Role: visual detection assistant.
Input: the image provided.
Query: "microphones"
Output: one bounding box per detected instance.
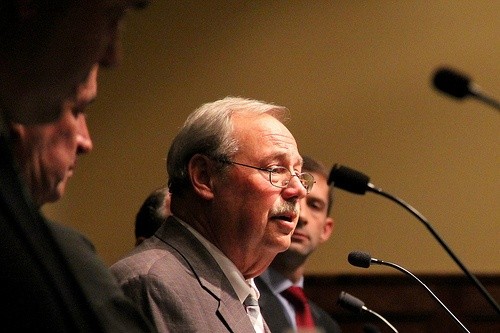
[336,290,400,333]
[432,66,500,114]
[347,249,471,333]
[326,163,500,314]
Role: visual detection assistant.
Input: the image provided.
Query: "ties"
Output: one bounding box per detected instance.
[279,286,316,333]
[243,287,265,333]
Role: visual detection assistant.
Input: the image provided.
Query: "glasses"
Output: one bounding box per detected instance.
[212,156,316,195]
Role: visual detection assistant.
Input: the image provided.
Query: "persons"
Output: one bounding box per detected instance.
[0,0,152,333]
[110,97,317,333]
[135,186,172,244]
[256,155,344,333]
